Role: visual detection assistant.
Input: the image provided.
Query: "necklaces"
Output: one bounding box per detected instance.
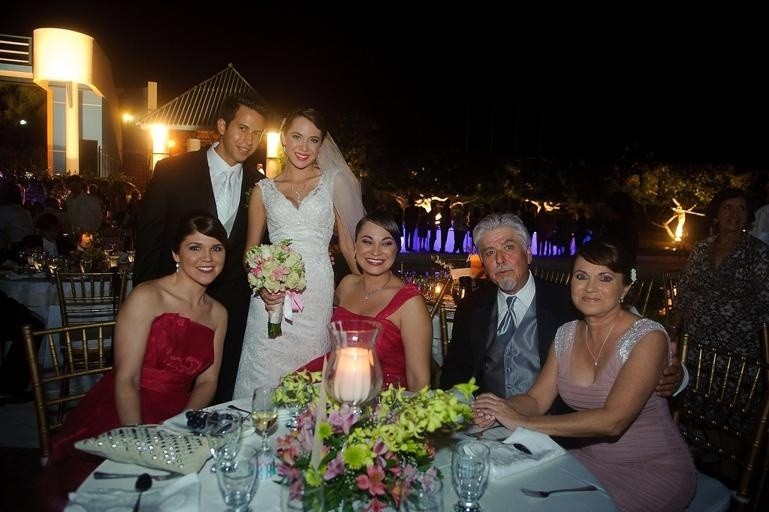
[585,318,617,367]
[362,275,391,299]
[284,176,309,204]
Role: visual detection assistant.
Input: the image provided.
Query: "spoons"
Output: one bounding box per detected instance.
[132,473,152,512]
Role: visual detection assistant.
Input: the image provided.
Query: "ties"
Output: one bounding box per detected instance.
[496,296,518,349]
[215,168,235,225]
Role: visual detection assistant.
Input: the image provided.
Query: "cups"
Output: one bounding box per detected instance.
[470,252,482,275]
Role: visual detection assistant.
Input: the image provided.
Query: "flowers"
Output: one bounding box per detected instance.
[245,239,306,339]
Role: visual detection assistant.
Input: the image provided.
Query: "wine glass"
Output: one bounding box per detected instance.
[205,386,278,512]
[280,470,325,512]
[451,439,491,512]
[400,274,463,305]
[16,250,27,274]
[27,250,136,277]
[399,471,446,511]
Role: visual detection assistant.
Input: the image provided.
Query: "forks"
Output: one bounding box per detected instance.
[521,485,599,499]
[94,471,183,482]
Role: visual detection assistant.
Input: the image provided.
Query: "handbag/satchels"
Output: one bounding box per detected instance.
[74,424,226,475]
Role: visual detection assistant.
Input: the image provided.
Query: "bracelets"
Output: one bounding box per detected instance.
[666,322,682,331]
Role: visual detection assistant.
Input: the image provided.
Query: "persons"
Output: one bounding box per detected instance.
[598,189,641,266]
[0,170,146,406]
[232,104,368,402]
[469,238,698,511]
[371,188,590,257]
[291,211,434,393]
[132,91,271,404]
[661,187,768,511]
[439,212,690,447]
[34,209,230,512]
